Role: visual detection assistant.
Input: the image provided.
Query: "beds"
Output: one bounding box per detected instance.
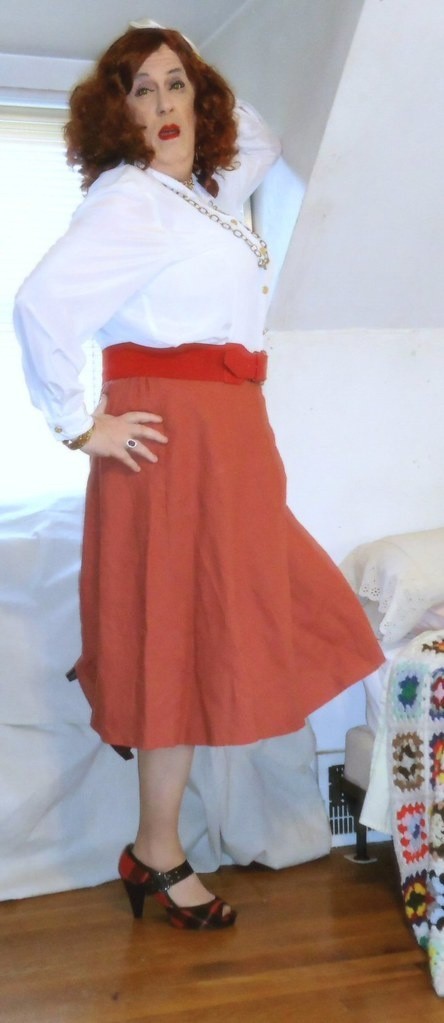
[341,629,444,999]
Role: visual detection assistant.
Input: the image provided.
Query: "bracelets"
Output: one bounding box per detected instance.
[63,424,94,451]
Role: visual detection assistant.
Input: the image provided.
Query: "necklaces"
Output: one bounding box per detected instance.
[161,183,269,269]
[181,179,194,189]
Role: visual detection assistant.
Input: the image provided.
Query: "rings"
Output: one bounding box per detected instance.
[127,439,137,449]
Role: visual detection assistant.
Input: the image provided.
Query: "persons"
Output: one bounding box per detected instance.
[12,20,386,930]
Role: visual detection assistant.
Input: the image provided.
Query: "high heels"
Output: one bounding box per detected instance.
[118,845,238,929]
[67,667,136,760]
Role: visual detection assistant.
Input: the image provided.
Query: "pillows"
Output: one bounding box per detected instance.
[338,529,444,646]
